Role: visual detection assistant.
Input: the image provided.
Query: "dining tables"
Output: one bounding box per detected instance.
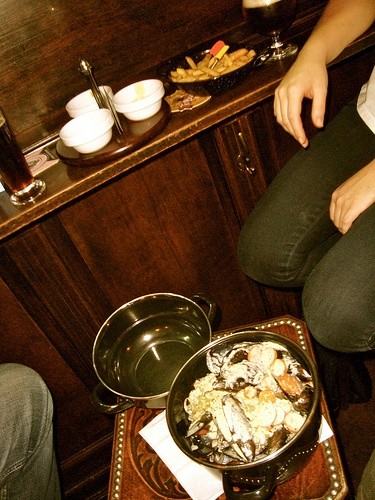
[104,314,349,500]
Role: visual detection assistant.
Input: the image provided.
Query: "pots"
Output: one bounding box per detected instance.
[87,292,217,415]
[155,41,270,97]
[166,327,321,500]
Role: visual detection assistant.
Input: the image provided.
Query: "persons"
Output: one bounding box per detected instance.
[238,0,375,352]
[0,363,62,500]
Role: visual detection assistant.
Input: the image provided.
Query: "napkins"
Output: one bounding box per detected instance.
[138,406,336,500]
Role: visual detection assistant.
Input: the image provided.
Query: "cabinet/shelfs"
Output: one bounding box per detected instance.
[0,4,375,500]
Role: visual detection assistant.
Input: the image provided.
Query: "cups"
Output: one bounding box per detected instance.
[0,105,46,205]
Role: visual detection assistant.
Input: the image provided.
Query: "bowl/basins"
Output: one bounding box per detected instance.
[112,79,165,121]
[65,85,114,118]
[58,109,115,154]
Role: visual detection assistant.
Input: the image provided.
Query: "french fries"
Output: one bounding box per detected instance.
[168,48,256,82]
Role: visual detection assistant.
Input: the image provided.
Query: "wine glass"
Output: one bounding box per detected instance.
[241,0,298,63]
